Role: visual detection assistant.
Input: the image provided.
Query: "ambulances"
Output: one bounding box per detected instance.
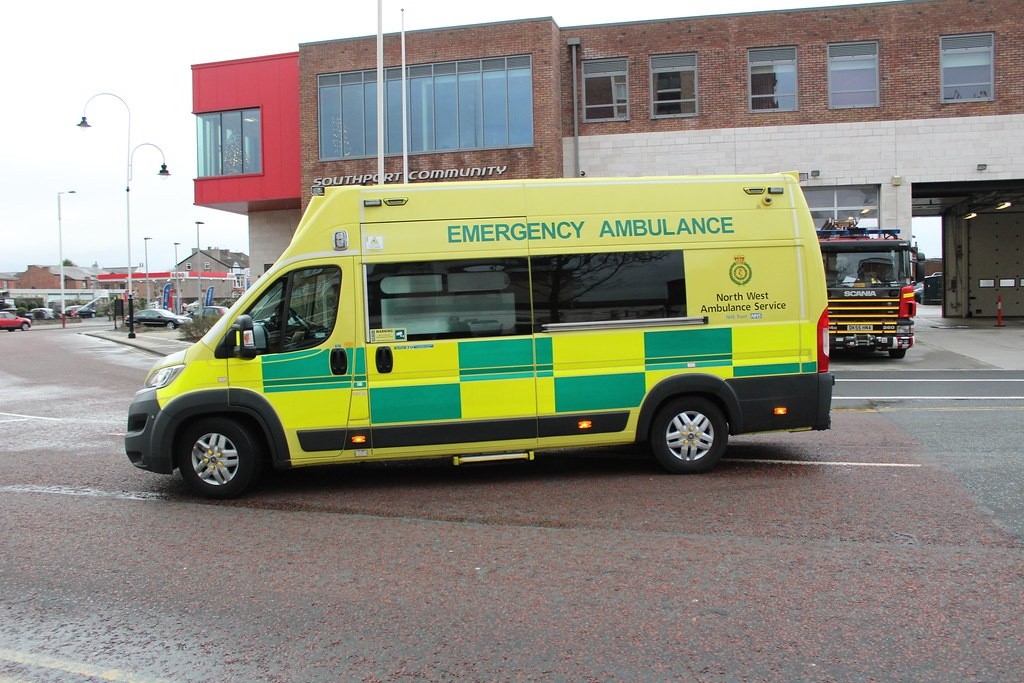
[124,171,834,497]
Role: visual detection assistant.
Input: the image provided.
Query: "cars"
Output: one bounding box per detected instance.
[125,309,193,331]
[915,272,942,303]
[0,312,31,332]
[63,305,96,318]
[30,308,55,320]
[921,276,942,305]
[181,306,230,324]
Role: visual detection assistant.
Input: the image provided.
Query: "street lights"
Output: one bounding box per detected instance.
[196,221,205,332]
[174,242,181,314]
[77,93,170,338]
[144,237,151,309]
[58,191,75,329]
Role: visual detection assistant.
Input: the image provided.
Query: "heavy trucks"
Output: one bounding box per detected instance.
[816,217,926,359]
